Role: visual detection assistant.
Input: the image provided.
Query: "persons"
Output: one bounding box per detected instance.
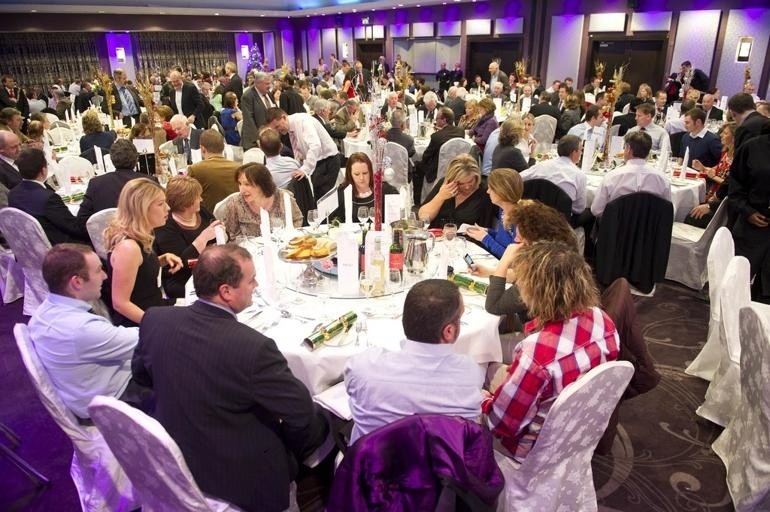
[119,244,330,511]
[27,242,140,426]
[479,244,620,459]
[339,279,486,446]
[1,54,770,328]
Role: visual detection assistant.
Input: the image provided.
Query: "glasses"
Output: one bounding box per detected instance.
[436,117,443,121]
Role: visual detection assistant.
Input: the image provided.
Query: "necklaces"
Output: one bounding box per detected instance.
[352,199,375,204]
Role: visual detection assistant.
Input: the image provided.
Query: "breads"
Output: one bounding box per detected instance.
[282,235,340,261]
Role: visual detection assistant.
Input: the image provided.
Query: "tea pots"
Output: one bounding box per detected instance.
[656,148,671,172]
[404,230,435,273]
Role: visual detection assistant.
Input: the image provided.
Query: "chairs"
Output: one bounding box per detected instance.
[0,245,25,304]
[10,322,144,510]
[87,395,301,511]
[46,112,93,185]
[289,175,313,222]
[495,359,636,511]
[85,207,139,260]
[328,412,504,512]
[512,179,586,278]
[376,142,408,197]
[0,206,65,317]
[668,197,770,512]
[420,138,476,204]
[596,193,673,298]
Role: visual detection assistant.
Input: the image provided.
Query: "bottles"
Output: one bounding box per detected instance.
[370,237,385,296]
[181,258,198,268]
[389,230,403,281]
[358,230,368,278]
[399,208,406,220]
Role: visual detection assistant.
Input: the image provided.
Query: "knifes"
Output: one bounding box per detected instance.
[338,323,352,345]
[243,310,263,325]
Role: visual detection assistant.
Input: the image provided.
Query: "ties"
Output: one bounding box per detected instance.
[184,138,192,165]
[120,87,135,114]
[9,89,14,97]
[262,94,271,109]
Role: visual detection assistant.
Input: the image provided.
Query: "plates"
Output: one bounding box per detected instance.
[457,284,481,295]
[318,324,357,347]
[672,181,689,187]
[312,260,338,276]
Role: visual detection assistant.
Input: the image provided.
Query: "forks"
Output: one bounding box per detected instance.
[259,317,281,337]
[361,320,369,347]
[354,321,362,346]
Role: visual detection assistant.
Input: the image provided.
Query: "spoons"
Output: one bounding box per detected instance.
[280,311,314,321]
[362,309,394,318]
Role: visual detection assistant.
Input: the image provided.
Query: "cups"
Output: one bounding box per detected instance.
[389,221,408,244]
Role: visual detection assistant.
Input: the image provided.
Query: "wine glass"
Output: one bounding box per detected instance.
[361,272,375,304]
[288,262,304,304]
[551,145,559,159]
[357,207,369,226]
[369,206,376,227]
[450,236,466,266]
[307,211,320,233]
[274,222,284,248]
[313,209,325,228]
[316,280,330,308]
[407,212,415,225]
[444,223,456,242]
[236,235,247,246]
[419,213,430,228]
[386,270,401,305]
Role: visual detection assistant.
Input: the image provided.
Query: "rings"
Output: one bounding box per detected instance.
[451,191,453,195]
